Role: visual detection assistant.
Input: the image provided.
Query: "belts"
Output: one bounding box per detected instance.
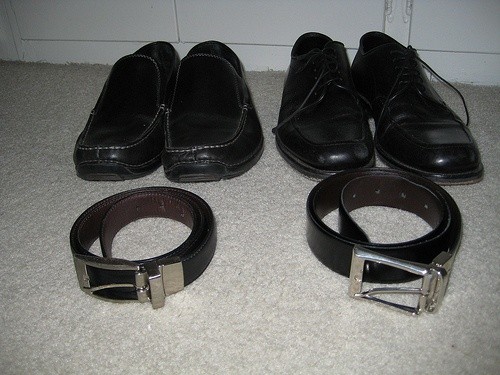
[70,186,218,309]
[305,167,464,317]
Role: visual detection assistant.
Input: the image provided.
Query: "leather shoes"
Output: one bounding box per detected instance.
[72,39,184,179]
[163,39,265,184]
[271,31,377,182]
[348,32,485,186]
